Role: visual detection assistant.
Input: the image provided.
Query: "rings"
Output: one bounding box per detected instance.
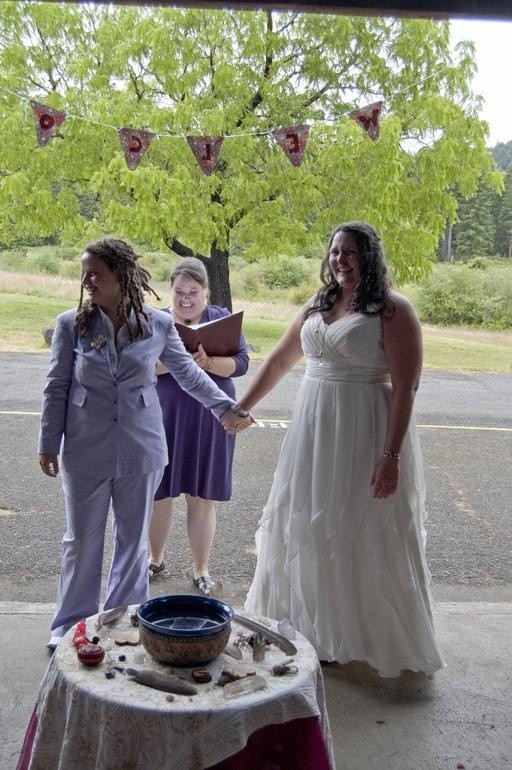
[385,486,393,492]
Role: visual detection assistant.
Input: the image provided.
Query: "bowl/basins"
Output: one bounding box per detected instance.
[136,594,234,668]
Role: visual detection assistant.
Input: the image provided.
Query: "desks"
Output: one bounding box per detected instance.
[17,605,335,767]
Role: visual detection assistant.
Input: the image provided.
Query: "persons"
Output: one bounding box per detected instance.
[147,257,250,595]
[37,236,253,650]
[221,219,447,680]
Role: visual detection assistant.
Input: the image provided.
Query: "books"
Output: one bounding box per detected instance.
[175,311,244,357]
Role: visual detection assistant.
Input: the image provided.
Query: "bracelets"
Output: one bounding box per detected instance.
[207,355,215,373]
[381,451,401,460]
[233,407,250,418]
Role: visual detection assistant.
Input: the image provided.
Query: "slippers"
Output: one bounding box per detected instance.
[148,561,166,580]
[192,576,218,596]
